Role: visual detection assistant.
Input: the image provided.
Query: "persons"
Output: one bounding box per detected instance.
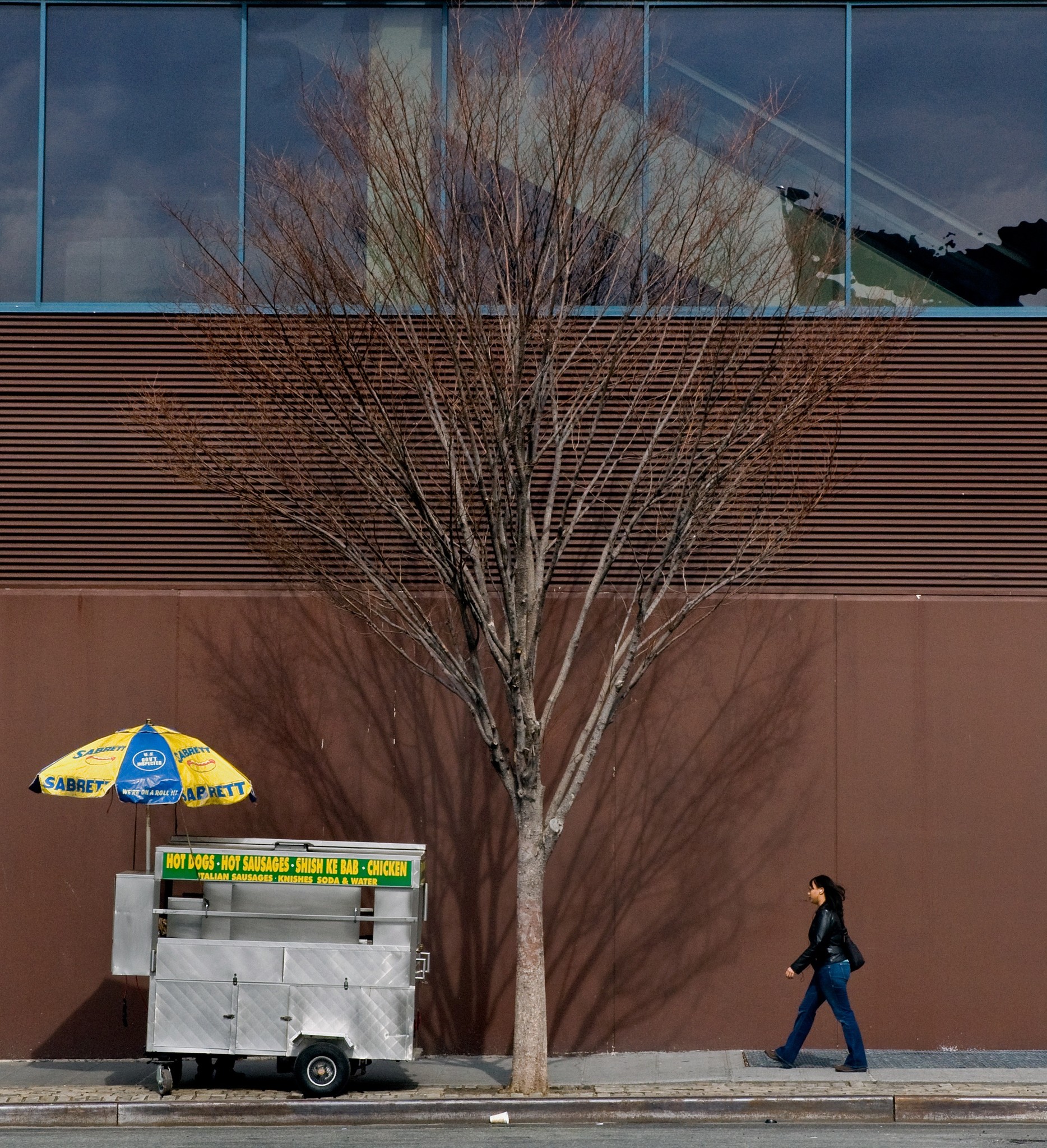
[764,875,868,1073]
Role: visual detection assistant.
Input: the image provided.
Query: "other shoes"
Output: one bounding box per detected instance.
[764,1047,793,1069]
[836,1065,867,1072]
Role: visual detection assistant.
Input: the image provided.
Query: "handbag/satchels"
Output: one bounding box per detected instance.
[844,934,865,973]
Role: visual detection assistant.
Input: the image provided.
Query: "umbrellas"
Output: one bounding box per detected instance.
[27,718,257,872]
